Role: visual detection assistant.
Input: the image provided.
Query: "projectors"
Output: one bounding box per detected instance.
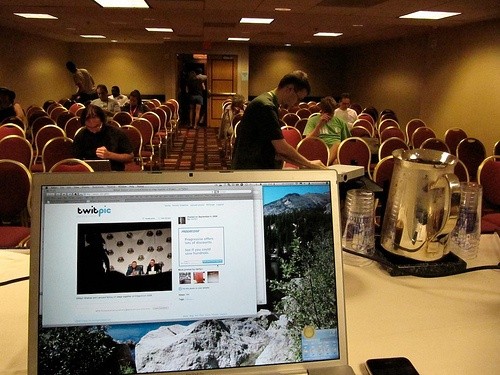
[325,164,365,182]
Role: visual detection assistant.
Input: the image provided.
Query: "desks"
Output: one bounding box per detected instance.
[0,233,500,375]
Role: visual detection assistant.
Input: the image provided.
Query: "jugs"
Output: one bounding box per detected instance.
[379,148,462,262]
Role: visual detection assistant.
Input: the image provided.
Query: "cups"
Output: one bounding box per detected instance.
[450,181,482,262]
[341,188,376,267]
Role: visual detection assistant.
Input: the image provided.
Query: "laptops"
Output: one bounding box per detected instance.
[25,168,356,375]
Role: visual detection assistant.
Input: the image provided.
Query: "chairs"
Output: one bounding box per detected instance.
[217,99,500,237]
[156,263,163,273]
[130,265,143,274]
[0,94,179,251]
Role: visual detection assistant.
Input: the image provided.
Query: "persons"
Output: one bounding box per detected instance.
[65,61,99,102]
[146,259,162,276]
[125,261,143,277]
[182,65,209,129]
[71,105,134,172]
[334,95,358,129]
[121,89,149,117]
[0,87,24,124]
[231,72,327,170]
[303,96,352,167]
[79,231,112,293]
[89,84,122,116]
[107,87,128,105]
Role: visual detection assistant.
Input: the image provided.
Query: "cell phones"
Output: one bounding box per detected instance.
[366,356,420,375]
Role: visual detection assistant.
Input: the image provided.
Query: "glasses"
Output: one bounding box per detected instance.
[85,122,103,129]
[295,91,302,103]
